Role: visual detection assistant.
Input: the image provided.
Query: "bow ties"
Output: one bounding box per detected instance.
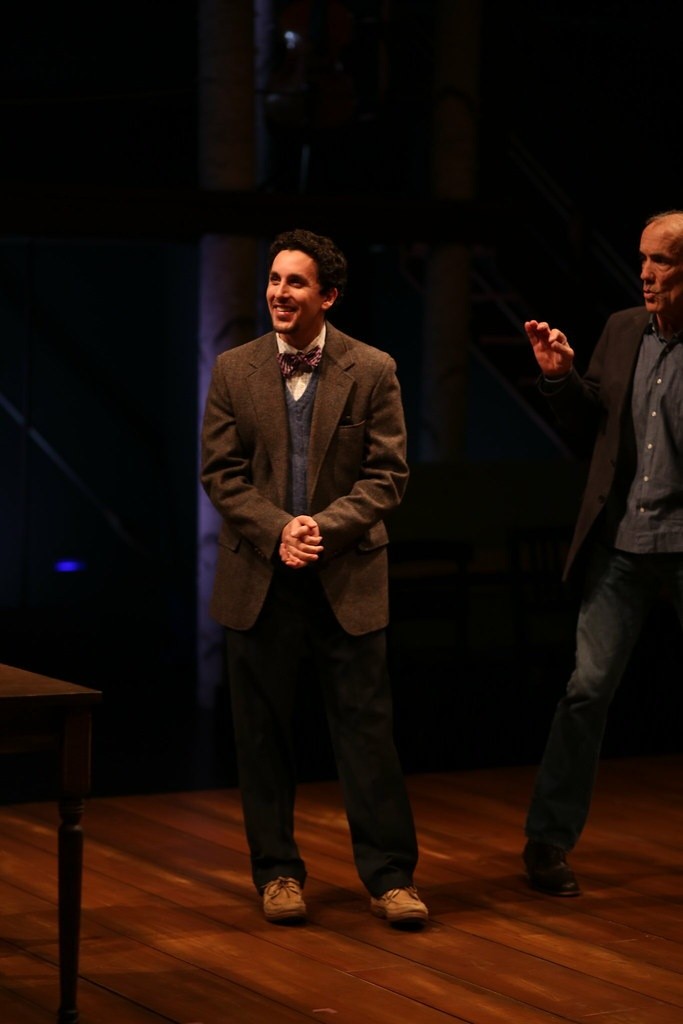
[275,348,322,380]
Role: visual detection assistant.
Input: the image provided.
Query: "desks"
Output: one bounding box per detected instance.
[0,663,103,1024]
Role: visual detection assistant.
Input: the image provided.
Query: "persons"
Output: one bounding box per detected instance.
[199,231,435,927]
[524,211,683,898]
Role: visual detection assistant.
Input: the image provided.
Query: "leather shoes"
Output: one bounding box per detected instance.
[263,877,307,922]
[368,888,429,924]
[525,856,581,897]
